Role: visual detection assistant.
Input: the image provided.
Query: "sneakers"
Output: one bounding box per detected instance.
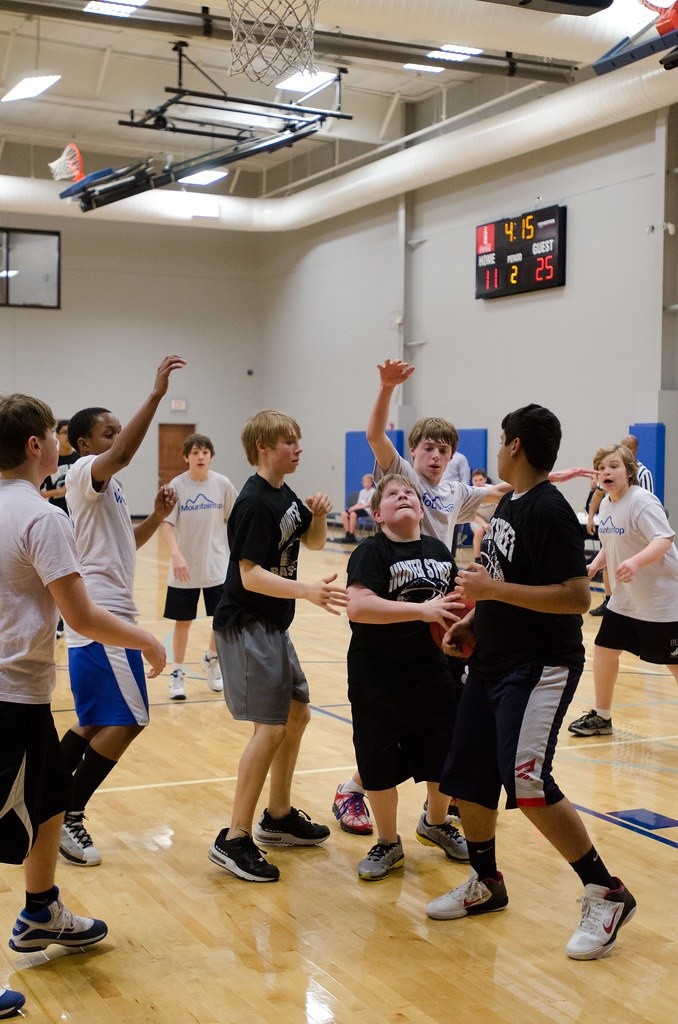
[201,651,224,692]
[566,877,636,961]
[168,669,187,699]
[0,986,25,1017]
[254,806,331,846]
[9,900,108,954]
[567,709,614,736]
[422,795,460,818]
[356,834,405,882]
[207,827,280,882]
[425,870,508,920]
[59,808,102,866]
[332,782,374,834]
[416,812,469,861]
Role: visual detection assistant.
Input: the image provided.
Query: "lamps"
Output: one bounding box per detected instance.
[176,129,230,186]
[0,16,61,103]
[274,63,339,95]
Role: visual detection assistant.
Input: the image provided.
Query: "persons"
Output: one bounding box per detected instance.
[345,403,637,960]
[206,410,351,883]
[568,434,678,737]
[162,433,240,701]
[60,354,188,866]
[39,419,81,639]
[440,450,471,558]
[341,474,376,543]
[0,392,167,1019]
[331,355,600,836]
[468,467,499,565]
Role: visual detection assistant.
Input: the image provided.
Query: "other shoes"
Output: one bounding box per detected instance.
[341,534,356,543]
[588,600,609,616]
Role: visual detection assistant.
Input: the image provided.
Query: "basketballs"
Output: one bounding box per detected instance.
[431,590,475,658]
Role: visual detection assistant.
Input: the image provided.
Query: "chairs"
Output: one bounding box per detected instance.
[327,491,378,543]
